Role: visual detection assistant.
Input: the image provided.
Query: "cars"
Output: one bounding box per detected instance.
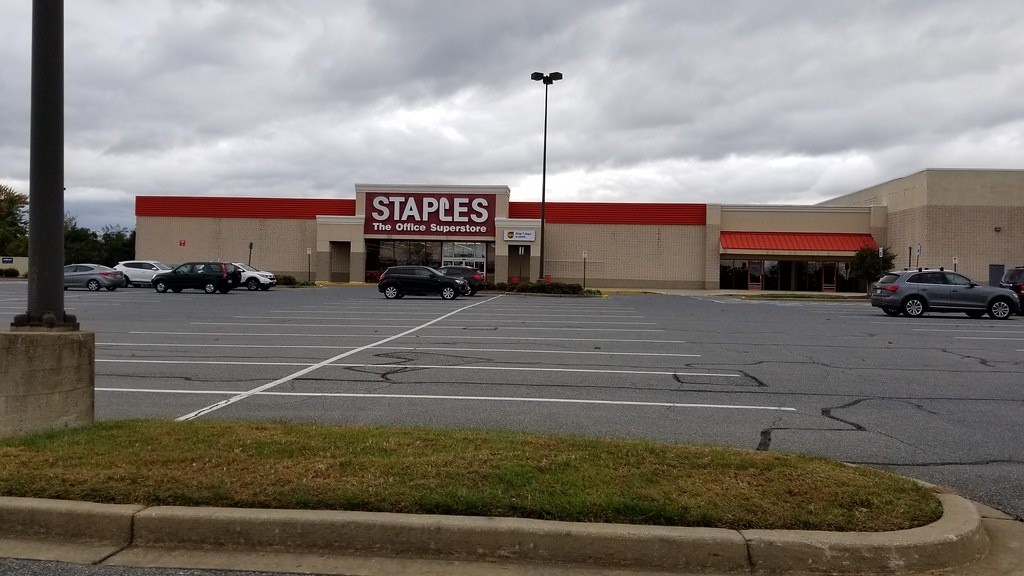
[871,266,1020,320]
[64,264,126,292]
[113,261,173,287]
[164,264,190,274]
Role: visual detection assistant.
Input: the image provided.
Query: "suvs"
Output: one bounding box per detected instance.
[378,265,469,300]
[198,264,277,291]
[151,262,237,294]
[1000,267,1024,315]
[434,265,486,296]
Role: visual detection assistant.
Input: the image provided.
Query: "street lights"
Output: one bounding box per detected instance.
[532,71,563,279]
